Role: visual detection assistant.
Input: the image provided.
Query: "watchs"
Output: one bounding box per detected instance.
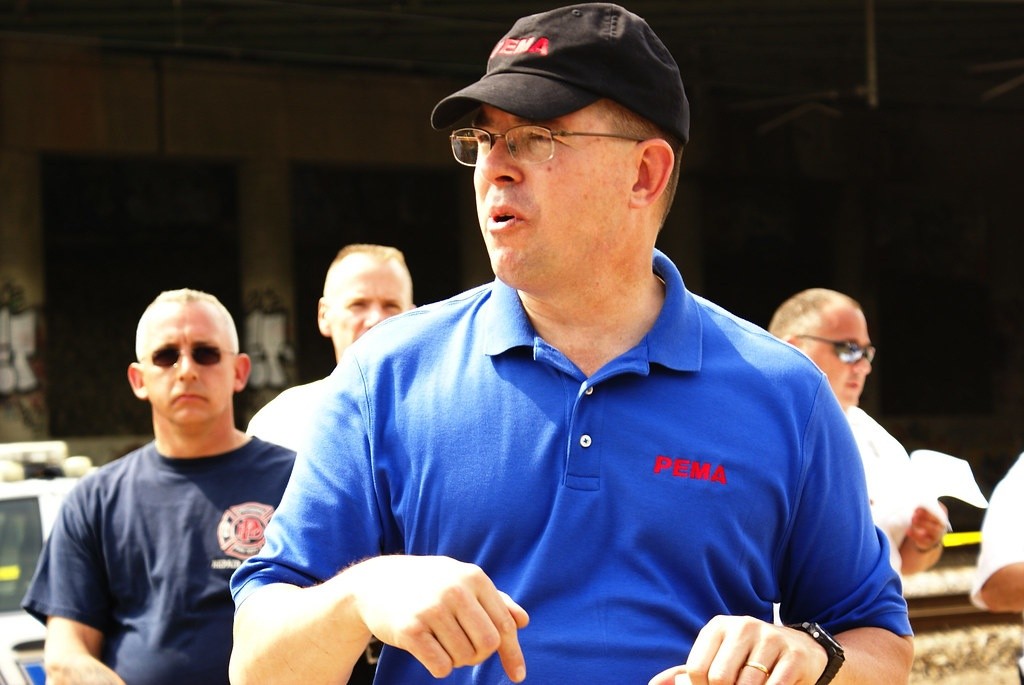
[787,620,845,685]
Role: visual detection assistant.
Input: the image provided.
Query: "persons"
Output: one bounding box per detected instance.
[22,289,296,685]
[227,0,913,685]
[768,288,948,578]
[246,245,417,685]
[970,452,1023,611]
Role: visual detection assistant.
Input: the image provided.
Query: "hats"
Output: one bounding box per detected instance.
[430,3,690,133]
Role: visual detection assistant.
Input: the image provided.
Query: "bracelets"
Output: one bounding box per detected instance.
[916,541,941,552]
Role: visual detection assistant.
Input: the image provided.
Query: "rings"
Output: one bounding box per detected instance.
[745,659,770,674]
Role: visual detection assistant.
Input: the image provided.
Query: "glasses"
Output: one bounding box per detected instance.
[150,345,226,367]
[797,334,876,364]
[450,126,646,167]
